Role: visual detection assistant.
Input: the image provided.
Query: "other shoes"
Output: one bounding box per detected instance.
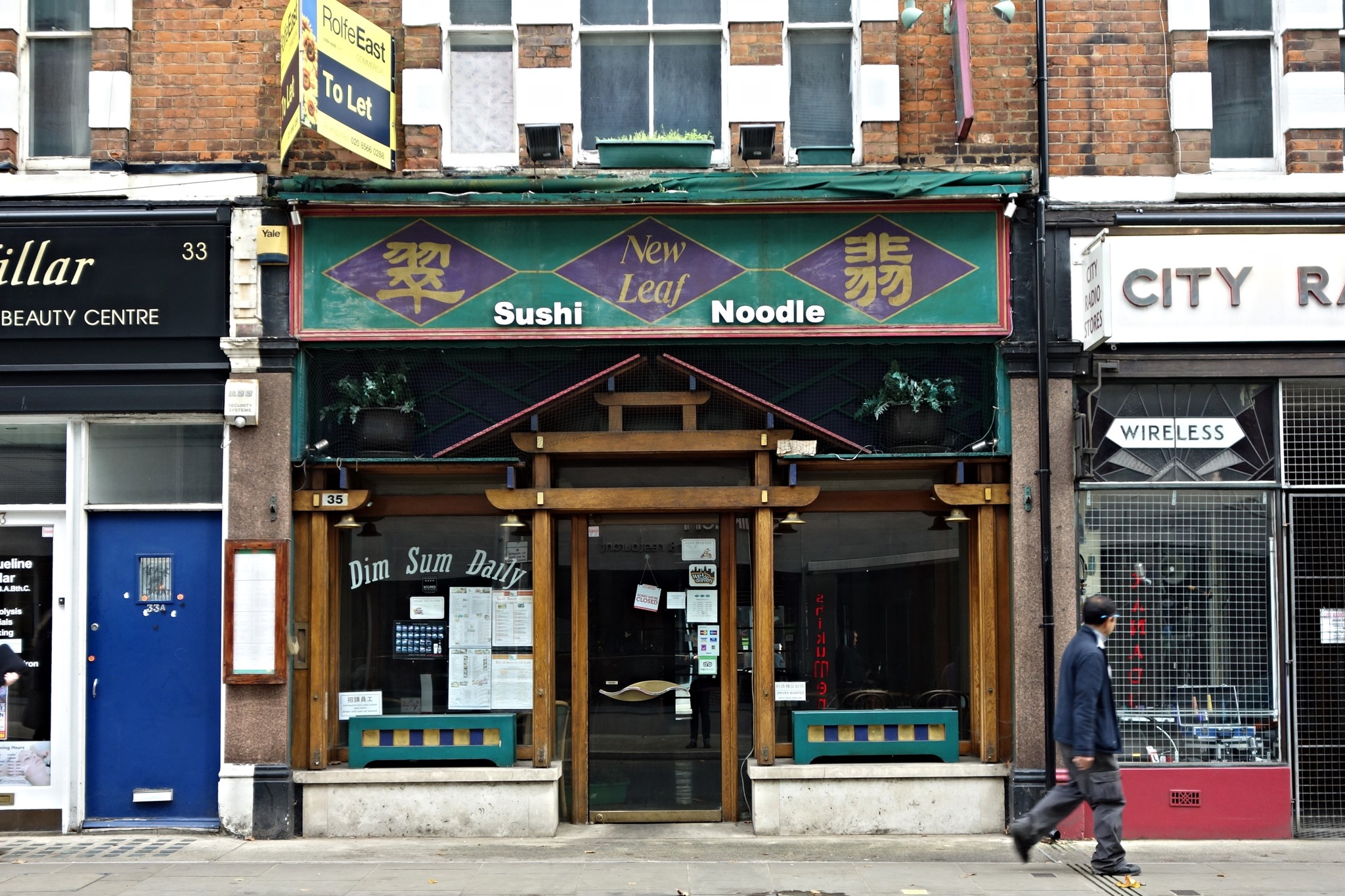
[704,743,711,749]
[686,742,698,749]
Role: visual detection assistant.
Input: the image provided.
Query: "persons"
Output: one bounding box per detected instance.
[2,672,21,686]
[1008,592,1143,876]
[937,646,961,691]
[10,749,50,786]
[834,629,882,710]
[30,610,52,741]
[774,652,786,672]
[0,742,51,778]
[684,649,712,749]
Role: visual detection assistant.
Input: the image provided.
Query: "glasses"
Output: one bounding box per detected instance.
[1101,614,1120,622]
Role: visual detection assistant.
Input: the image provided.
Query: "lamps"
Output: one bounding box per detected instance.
[991,0,1015,24]
[900,0,925,31]
[524,124,565,162]
[737,123,776,160]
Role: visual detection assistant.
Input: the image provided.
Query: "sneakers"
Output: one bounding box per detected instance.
[1110,863,1142,877]
[1009,822,1030,863]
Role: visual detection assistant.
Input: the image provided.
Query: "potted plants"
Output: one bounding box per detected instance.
[315,356,428,459]
[595,124,716,168]
[855,359,970,453]
